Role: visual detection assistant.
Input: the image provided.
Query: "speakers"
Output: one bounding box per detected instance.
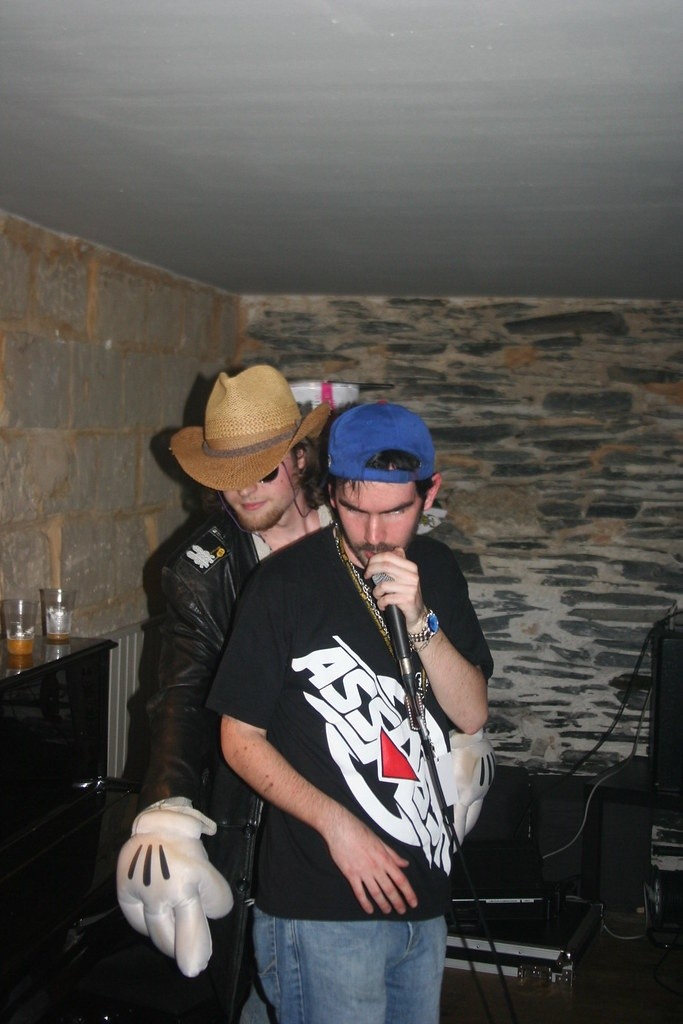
[649,825,683,934]
[649,630,683,793]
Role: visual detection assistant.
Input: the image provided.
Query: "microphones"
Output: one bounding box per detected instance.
[373,573,421,719]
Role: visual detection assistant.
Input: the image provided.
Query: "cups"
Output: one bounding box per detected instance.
[3,599,39,655]
[45,588,76,644]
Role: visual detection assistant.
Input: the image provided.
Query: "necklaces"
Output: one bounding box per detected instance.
[334,521,426,731]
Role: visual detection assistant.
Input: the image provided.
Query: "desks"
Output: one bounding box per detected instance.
[582,756,683,899]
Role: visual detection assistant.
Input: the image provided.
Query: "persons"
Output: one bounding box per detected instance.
[205,399,495,1024]
[116,365,331,1024]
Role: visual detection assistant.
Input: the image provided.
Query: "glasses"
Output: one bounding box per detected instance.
[258,465,278,482]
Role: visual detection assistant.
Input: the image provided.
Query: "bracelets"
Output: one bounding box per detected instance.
[410,640,430,652]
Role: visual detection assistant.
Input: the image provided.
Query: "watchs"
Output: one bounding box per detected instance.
[409,607,439,642]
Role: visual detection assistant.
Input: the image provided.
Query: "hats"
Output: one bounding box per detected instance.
[169,364,328,490]
[328,401,435,482]
